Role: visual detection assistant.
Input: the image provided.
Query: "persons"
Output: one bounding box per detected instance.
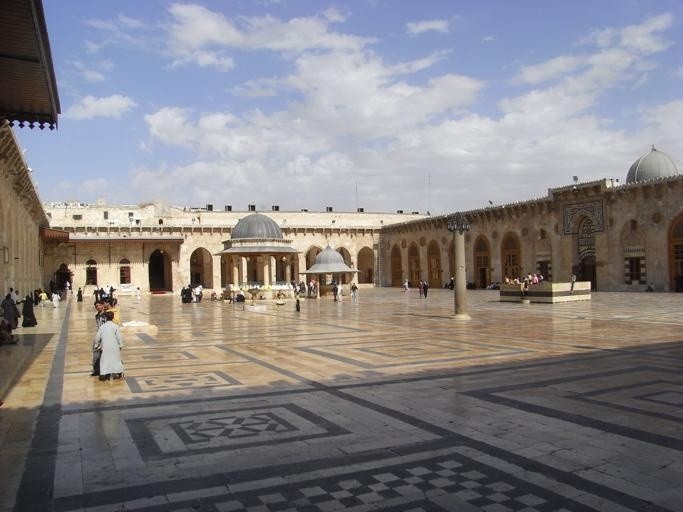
[569,273,577,293]
[0,280,141,381]
[181,279,358,311]
[403,273,543,299]
